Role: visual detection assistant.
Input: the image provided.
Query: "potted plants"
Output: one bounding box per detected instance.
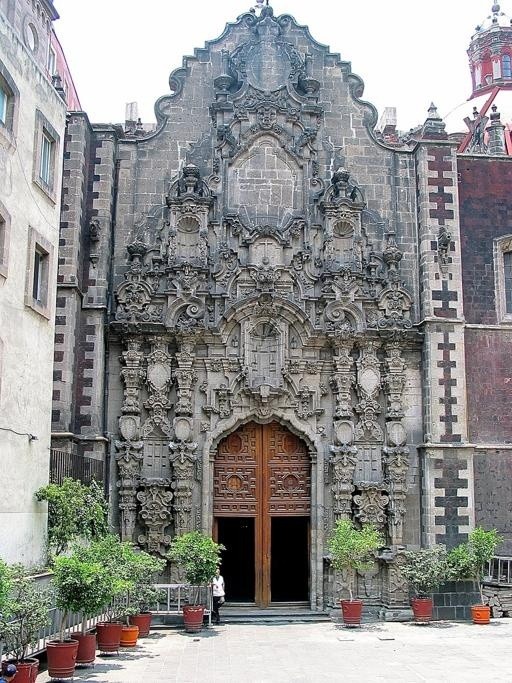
[323,515,505,629]
[0,530,229,683]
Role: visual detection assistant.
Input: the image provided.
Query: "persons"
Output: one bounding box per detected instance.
[211,569,225,624]
